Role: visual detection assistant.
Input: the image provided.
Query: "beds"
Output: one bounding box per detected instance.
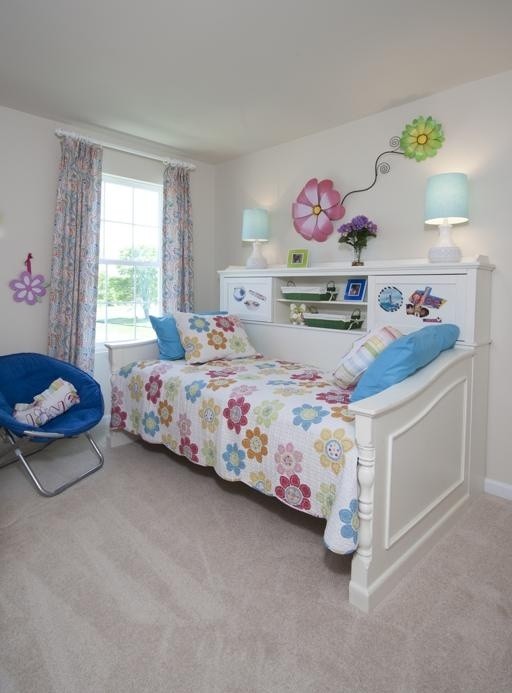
[102,313,481,610]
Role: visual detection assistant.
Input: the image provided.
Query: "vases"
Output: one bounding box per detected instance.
[351,259,364,266]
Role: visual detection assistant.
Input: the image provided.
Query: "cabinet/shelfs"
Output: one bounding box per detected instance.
[218,268,490,343]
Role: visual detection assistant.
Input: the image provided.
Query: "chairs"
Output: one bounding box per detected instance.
[0,353,107,496]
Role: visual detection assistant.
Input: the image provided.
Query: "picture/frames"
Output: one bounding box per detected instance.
[344,279,367,301]
[287,248,309,269]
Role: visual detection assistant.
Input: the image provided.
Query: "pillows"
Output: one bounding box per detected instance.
[147,315,259,364]
[328,321,458,399]
[11,374,81,430]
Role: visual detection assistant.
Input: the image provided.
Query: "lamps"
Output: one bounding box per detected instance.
[424,174,473,262]
[239,208,271,270]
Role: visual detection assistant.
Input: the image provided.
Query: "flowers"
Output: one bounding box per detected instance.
[337,215,379,261]
[288,303,308,326]
